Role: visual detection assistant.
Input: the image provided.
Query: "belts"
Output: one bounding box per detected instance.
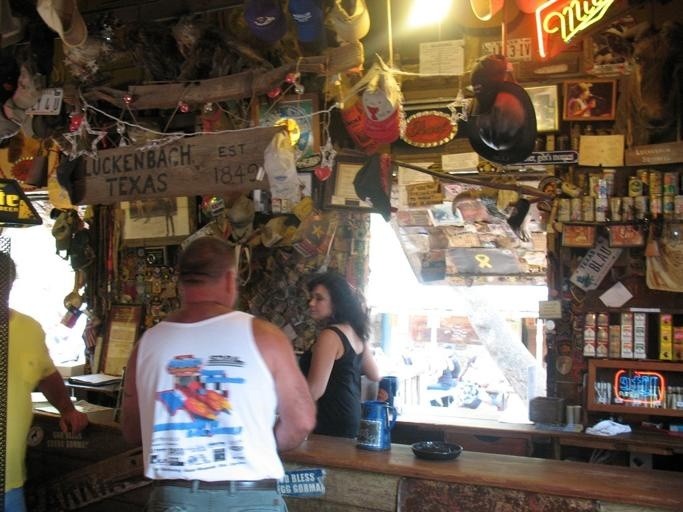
[153,478,277,491]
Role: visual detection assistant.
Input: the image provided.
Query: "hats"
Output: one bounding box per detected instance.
[468,83,538,164]
[52,212,71,250]
[12,61,46,108]
[227,196,255,242]
[243,0,286,44]
[36,0,88,48]
[330,0,370,42]
[57,157,82,205]
[363,70,402,141]
[571,83,593,96]
[353,155,391,221]
[288,0,322,43]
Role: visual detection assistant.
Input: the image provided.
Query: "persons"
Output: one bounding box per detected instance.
[119,236,317,512]
[298,270,382,437]
[0,250,89,512]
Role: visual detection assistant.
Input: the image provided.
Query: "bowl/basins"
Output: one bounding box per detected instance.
[412,441,463,460]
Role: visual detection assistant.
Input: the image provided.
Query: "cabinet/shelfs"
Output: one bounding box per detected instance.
[555,218,683,470]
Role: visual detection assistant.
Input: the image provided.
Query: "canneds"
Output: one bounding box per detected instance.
[553,168,683,222]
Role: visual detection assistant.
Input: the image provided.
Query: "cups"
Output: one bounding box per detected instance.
[356,401,397,450]
[567,405,582,424]
